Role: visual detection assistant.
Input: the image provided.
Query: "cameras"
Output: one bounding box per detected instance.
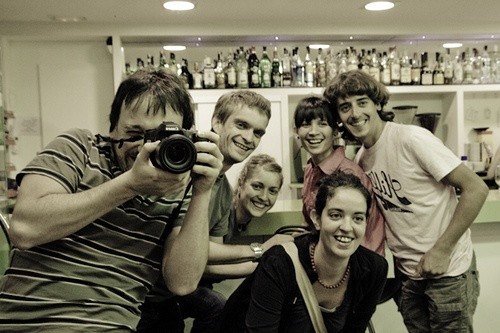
[143,121,211,174]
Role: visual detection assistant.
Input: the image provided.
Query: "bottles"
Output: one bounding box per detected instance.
[446,44,500,84]
[122,41,193,89]
[388,43,446,85]
[282,44,328,87]
[193,46,283,88]
[327,46,390,87]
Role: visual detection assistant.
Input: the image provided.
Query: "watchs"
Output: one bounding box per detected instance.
[250,242,263,258]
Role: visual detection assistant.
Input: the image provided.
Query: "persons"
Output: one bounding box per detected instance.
[246,168,388,333]
[324,70,489,333]
[176,91,295,333]
[0,66,224,333]
[294,97,386,261]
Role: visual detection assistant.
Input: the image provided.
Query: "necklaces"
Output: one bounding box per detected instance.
[311,243,350,288]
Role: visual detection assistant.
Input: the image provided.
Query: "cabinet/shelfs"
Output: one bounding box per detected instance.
[0,106,9,209]
[181,83,500,235]
